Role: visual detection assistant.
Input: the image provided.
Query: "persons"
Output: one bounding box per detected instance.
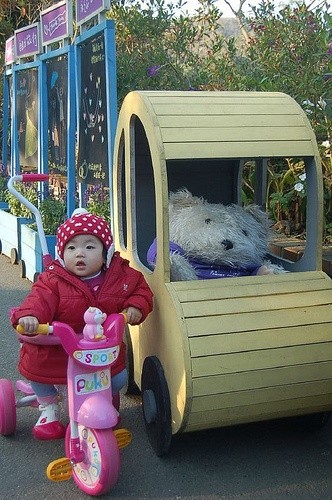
[11,209,153,427]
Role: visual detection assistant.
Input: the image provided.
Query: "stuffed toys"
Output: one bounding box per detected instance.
[148,189,287,282]
